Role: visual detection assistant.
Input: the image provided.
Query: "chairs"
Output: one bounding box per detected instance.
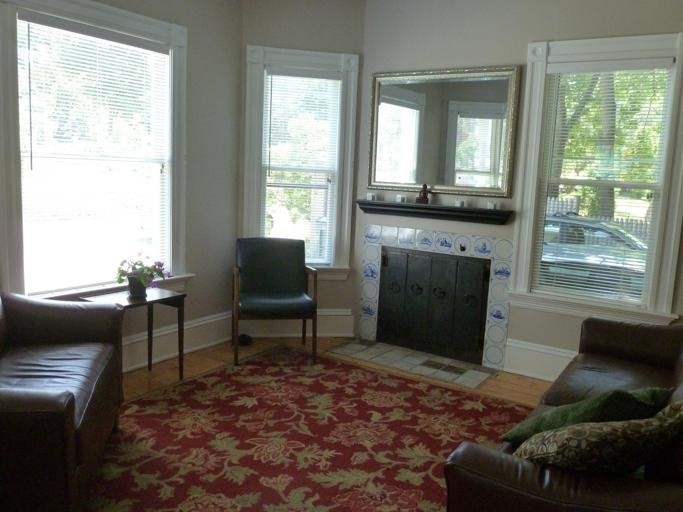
[574,229,585,244]
[233,237,318,365]
[567,231,577,244]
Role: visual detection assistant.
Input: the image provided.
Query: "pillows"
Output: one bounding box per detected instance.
[513,401,683,480]
[501,387,676,444]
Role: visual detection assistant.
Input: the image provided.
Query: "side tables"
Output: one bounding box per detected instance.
[79,287,187,380]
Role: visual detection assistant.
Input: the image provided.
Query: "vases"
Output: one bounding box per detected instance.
[129,275,146,298]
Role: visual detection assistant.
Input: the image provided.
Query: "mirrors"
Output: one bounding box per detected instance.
[366,65,521,199]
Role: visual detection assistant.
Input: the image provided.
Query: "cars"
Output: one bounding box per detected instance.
[537,214,648,292]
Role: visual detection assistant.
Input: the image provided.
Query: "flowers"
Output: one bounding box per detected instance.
[117,259,174,288]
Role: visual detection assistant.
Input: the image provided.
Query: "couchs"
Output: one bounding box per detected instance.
[445,318,683,512]
[0,291,124,512]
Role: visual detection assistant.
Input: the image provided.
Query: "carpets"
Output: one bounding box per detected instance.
[81,344,536,512]
